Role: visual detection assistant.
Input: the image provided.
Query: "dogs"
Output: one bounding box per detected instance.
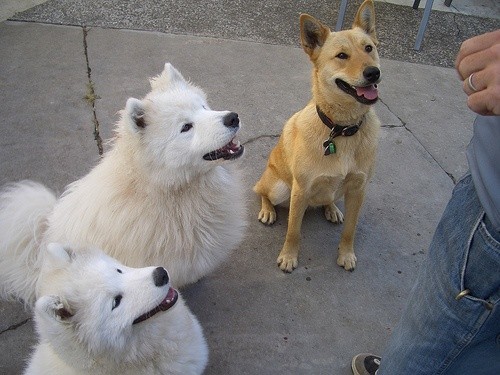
[24,242,210,374]
[250,0,383,275]
[1,62,248,309]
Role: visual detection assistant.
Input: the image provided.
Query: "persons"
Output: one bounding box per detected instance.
[351,29,500,375]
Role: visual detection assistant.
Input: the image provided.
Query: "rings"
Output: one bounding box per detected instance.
[468,72,479,92]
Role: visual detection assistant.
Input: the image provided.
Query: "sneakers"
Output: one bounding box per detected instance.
[351,353,382,375]
